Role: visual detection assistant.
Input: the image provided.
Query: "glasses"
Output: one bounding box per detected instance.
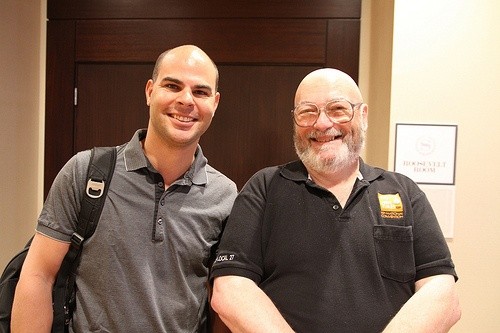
[291,99,362,127]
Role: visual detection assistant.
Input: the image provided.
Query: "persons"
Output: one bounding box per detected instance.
[207,68,462,332]
[8,44,240,332]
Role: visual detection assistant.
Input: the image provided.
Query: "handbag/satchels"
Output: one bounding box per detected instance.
[0,146,117,333]
